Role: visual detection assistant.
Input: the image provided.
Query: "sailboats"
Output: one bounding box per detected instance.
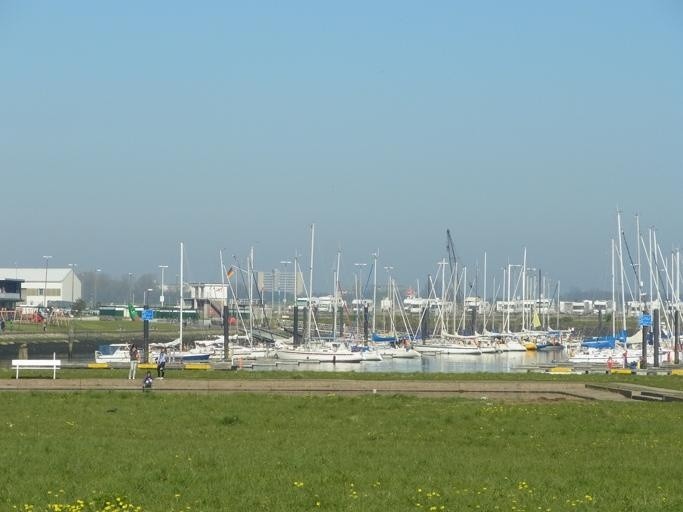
[569,212,681,363]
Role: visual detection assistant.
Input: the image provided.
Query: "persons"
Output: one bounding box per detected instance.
[129,344,139,380]
[143,371,154,388]
[607,356,612,376]
[155,348,167,379]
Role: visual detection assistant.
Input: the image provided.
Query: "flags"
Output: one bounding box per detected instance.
[228,265,238,279]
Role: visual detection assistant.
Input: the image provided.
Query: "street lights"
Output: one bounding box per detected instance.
[70,264,77,304]
[42,256,51,308]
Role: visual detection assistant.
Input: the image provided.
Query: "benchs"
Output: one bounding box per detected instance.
[11,359,62,381]
